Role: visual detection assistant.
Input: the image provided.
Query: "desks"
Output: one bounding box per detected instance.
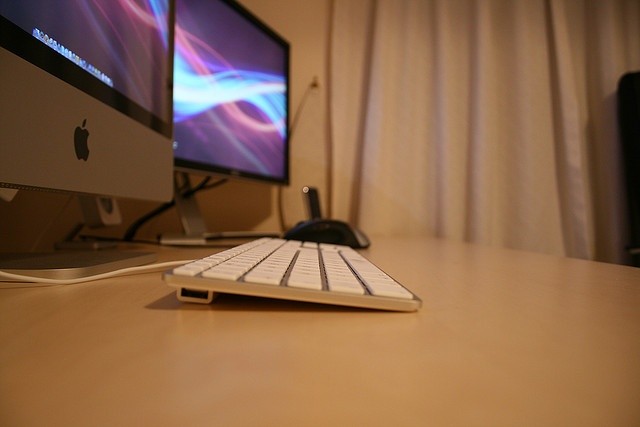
[1,229,639,426]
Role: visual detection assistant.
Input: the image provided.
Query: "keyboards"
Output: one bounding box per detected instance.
[161,236,422,313]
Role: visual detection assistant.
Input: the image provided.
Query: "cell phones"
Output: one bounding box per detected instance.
[302,186,320,220]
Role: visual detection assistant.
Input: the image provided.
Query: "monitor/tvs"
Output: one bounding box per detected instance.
[0,0,175,281]
[159,0,290,247]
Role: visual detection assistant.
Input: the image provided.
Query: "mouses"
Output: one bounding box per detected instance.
[284,218,371,250]
